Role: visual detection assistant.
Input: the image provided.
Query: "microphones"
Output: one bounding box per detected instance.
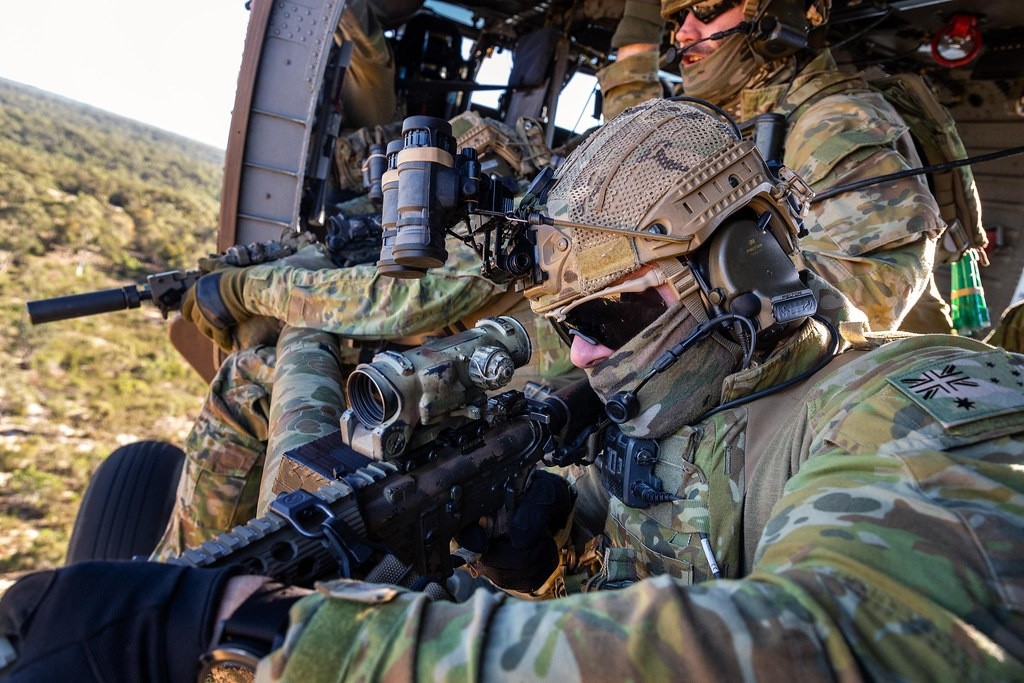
[665,31,723,65]
[603,325,710,423]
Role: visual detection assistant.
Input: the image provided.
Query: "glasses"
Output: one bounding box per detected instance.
[543,267,667,352]
[673,0,749,30]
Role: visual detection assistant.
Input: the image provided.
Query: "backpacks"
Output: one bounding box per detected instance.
[850,64,991,273]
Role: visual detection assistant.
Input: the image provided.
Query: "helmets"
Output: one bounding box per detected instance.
[521,98,810,320]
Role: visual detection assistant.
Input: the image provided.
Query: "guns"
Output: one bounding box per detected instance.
[169,314,606,595]
[25,212,383,328]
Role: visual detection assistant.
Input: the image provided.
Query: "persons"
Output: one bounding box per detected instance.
[147,0,516,560]
[595,0,959,336]
[0,94,1024,683]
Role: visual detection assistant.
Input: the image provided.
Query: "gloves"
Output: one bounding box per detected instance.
[180,257,255,354]
[0,558,256,682]
[455,469,581,592]
[610,1,668,48]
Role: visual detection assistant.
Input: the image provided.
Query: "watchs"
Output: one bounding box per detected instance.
[198,579,317,683]
